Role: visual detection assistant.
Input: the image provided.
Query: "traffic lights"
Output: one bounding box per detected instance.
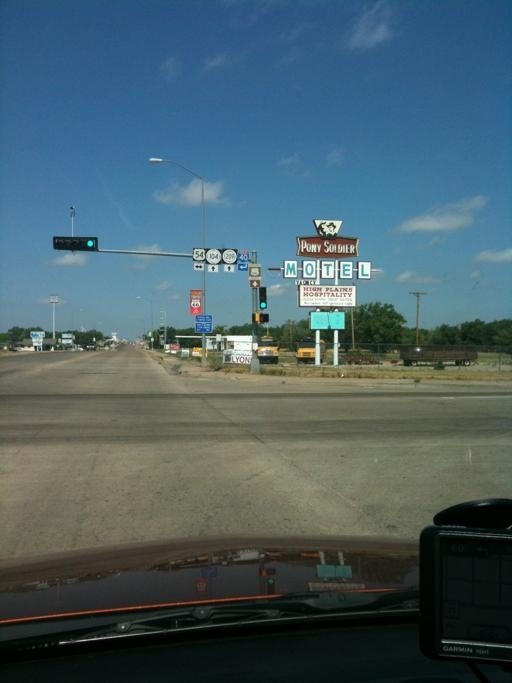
[267,577,275,594]
[259,286,268,310]
[256,312,270,323]
[259,568,268,577]
[53,236,98,252]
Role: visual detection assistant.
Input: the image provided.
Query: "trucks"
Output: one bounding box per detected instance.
[60,334,75,349]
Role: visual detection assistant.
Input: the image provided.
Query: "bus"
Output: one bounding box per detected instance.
[264,548,288,562]
[296,337,326,364]
[300,549,328,565]
[255,336,280,364]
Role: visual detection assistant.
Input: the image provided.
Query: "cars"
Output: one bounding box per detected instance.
[86,343,97,351]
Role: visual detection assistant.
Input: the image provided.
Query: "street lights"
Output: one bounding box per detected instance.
[149,158,206,368]
[136,295,154,351]
